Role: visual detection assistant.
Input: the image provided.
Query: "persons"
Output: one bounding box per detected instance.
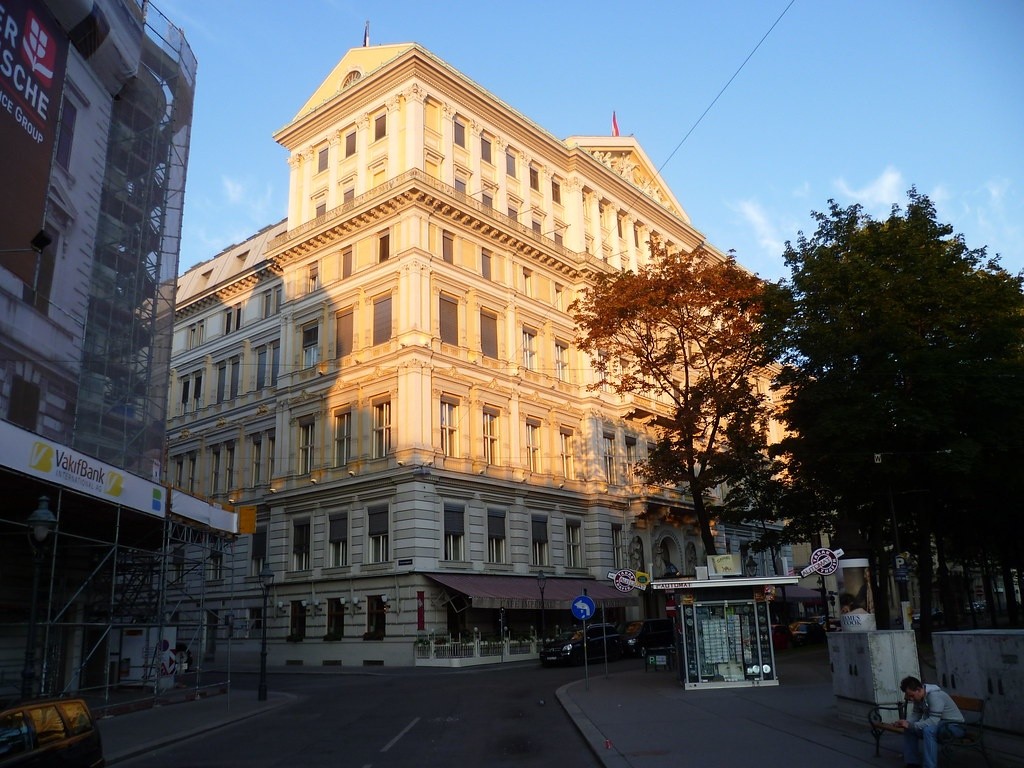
[890,675,967,768]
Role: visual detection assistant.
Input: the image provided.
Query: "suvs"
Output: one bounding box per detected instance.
[539,622,619,668]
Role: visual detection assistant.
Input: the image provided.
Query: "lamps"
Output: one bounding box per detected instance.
[381,596,390,608]
[302,601,310,612]
[278,602,286,613]
[340,597,348,611]
[314,600,322,611]
[353,597,361,609]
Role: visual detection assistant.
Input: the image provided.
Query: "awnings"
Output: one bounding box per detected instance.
[423,572,642,610]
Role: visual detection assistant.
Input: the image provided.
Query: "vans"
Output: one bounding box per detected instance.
[0,693,106,768]
[620,616,674,658]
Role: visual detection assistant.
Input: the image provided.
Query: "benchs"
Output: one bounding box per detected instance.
[867,693,992,768]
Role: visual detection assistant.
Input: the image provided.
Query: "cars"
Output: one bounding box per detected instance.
[771,614,840,652]
[911,598,989,623]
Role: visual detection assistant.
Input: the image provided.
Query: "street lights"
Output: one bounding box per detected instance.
[816,577,831,632]
[536,569,547,649]
[258,561,276,700]
[499,606,506,663]
